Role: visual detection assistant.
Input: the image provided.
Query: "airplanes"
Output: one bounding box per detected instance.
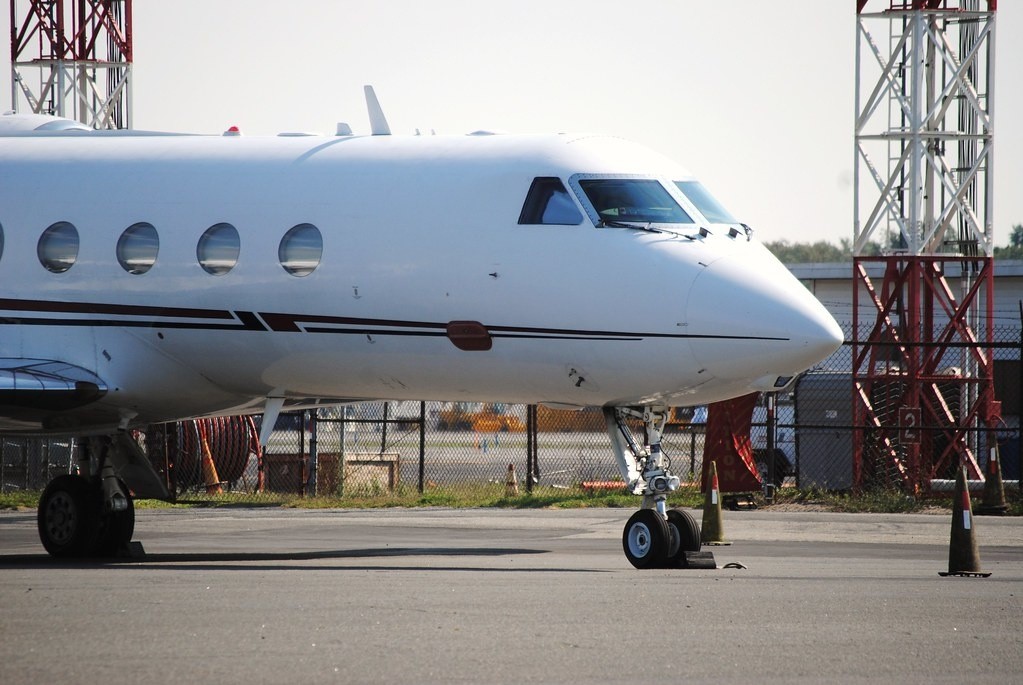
[0,85,844,570]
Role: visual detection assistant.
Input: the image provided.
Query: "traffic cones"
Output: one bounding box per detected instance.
[974,433,1011,514]
[696,460,733,546]
[198,437,228,495]
[939,466,996,580]
[503,462,520,500]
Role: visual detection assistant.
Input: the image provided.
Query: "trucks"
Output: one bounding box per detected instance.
[745,392,795,490]
